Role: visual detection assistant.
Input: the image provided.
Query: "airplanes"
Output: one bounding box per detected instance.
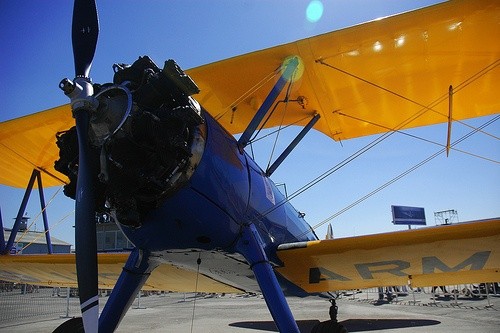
[1,1,500,333]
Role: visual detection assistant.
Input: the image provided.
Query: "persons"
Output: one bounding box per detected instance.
[0,281,40,293]
[432,286,451,298]
[52,287,61,296]
[378,286,422,301]
